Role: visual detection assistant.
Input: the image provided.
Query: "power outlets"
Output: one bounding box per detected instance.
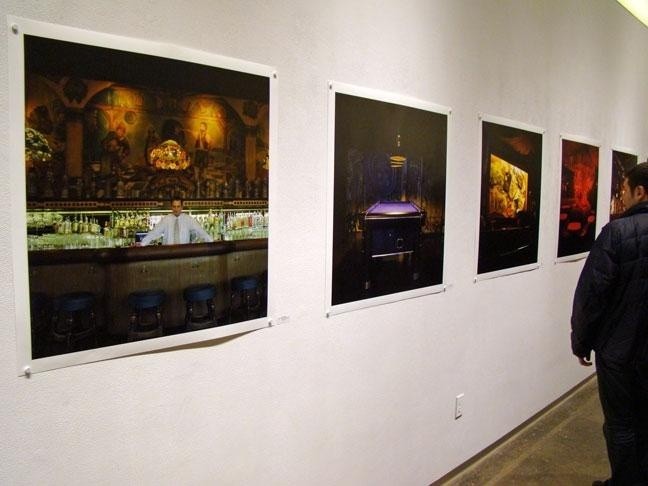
[455,394,465,420]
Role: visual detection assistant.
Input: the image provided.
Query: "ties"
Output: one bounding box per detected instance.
[174,217,180,244]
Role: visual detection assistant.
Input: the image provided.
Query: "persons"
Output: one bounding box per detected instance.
[186,124,215,168]
[571,162,646,485]
[100,123,132,170]
[132,198,221,246]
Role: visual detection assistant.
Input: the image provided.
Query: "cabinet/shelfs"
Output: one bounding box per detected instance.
[27,198,268,249]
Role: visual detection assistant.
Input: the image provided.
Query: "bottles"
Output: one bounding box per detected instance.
[195,173,202,199]
[122,223,126,237]
[116,173,125,199]
[225,210,268,227]
[126,182,195,200]
[27,214,110,237]
[111,227,148,238]
[111,208,151,227]
[226,228,268,239]
[203,176,268,200]
[194,210,224,240]
[26,166,99,200]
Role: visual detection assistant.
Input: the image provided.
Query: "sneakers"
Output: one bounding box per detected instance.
[592,477,614,485]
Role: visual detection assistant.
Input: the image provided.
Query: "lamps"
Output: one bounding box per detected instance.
[389,130,406,167]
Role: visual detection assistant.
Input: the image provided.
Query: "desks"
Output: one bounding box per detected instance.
[363,201,424,288]
